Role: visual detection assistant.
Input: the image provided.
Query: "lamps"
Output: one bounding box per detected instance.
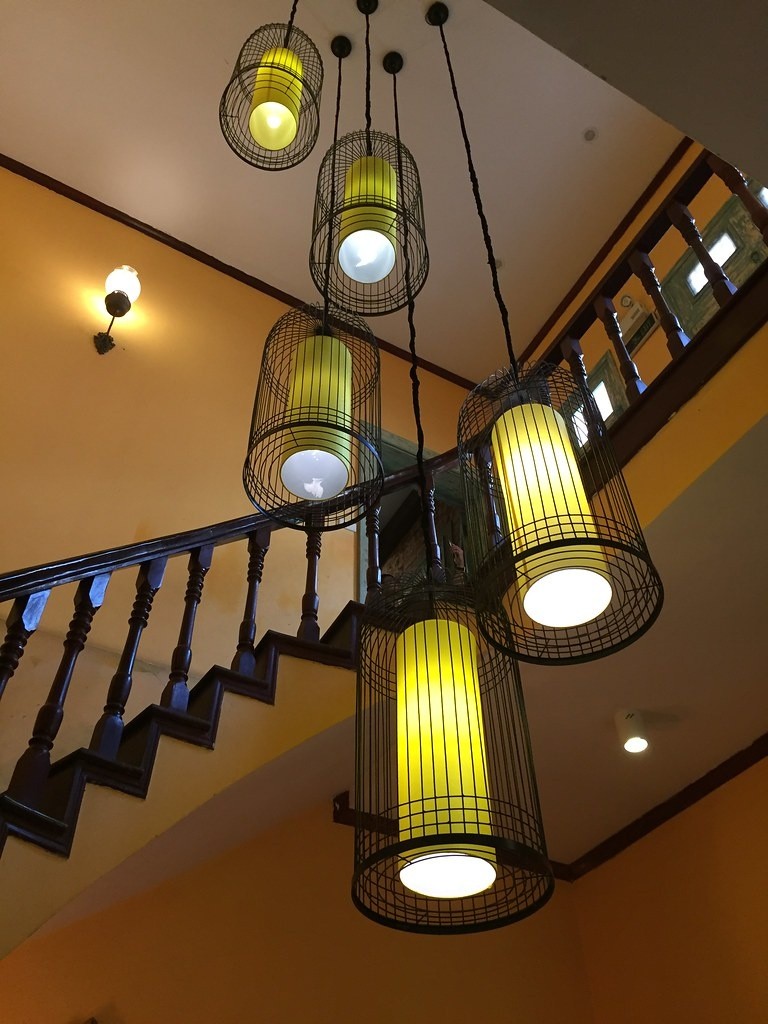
[94,264,141,355]
[307,16,429,317]
[614,709,652,760]
[437,25,666,667]
[349,71,558,936]
[241,54,385,532]
[218,0,324,171]
